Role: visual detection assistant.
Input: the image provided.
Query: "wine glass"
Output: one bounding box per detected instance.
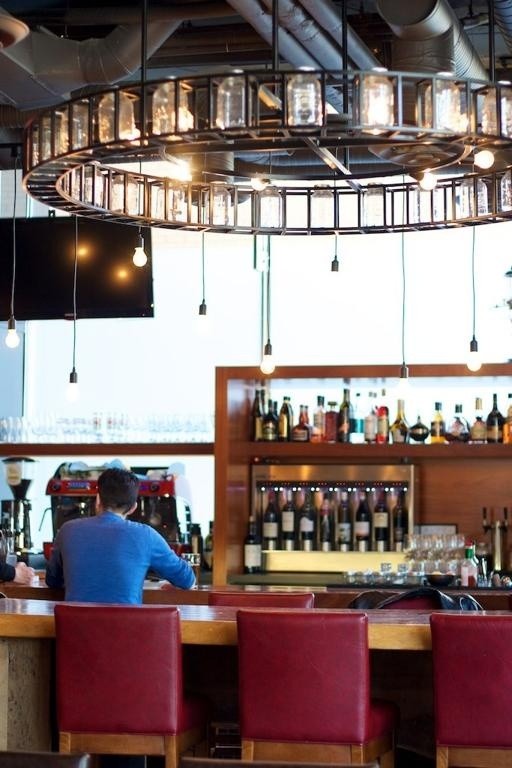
[402,532,468,576]
[0,409,214,445]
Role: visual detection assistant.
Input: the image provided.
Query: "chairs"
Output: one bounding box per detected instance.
[238,607,395,766]
[58,603,199,766]
[430,609,510,768]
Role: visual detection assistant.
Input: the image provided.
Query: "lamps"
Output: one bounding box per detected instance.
[130,168,152,275]
[330,141,350,298]
[253,238,276,379]
[462,182,488,373]
[63,212,87,403]
[193,199,216,333]
[4,92,25,354]
[393,168,422,402]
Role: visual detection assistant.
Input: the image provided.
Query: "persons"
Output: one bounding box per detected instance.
[43,467,195,608]
[0,558,36,587]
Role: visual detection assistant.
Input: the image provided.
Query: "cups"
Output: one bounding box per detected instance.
[343,561,464,589]
[182,554,202,588]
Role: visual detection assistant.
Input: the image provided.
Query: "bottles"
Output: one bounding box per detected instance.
[255,482,409,553]
[461,536,492,589]
[204,520,214,571]
[189,523,205,573]
[242,516,261,576]
[248,382,511,446]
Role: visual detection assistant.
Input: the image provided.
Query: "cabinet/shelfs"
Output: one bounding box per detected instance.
[213,362,511,592]
